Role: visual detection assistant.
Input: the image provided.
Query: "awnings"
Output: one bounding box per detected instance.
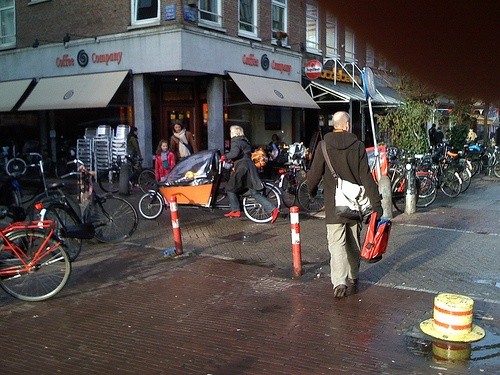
[224,70,320,109]
[0,80,33,111]
[18,71,129,110]
[312,79,409,133]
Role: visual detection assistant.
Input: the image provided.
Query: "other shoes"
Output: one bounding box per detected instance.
[350,278,359,284]
[333,285,347,298]
[271,207,280,224]
[224,210,241,217]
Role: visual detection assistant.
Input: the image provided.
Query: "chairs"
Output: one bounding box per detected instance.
[76,123,131,184]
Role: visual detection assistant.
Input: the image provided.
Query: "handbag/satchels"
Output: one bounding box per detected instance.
[335,177,366,220]
[268,143,279,160]
[178,138,194,156]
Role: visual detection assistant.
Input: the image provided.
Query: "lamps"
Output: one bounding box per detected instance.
[32,39,63,48]
[63,32,97,44]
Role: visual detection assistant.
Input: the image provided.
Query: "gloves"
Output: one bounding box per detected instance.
[374,209,383,220]
[220,156,226,161]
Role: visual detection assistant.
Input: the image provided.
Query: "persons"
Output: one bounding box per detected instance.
[269,134,281,149]
[429,123,444,152]
[468,127,477,141]
[374,117,380,144]
[219,125,280,223]
[155,139,176,208]
[305,111,383,297]
[127,127,143,187]
[170,119,198,161]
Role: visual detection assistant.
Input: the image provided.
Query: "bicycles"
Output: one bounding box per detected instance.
[0,205,72,302]
[278,136,500,213]
[138,157,283,224]
[0,153,156,262]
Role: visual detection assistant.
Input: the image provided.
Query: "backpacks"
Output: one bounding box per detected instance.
[360,212,392,263]
